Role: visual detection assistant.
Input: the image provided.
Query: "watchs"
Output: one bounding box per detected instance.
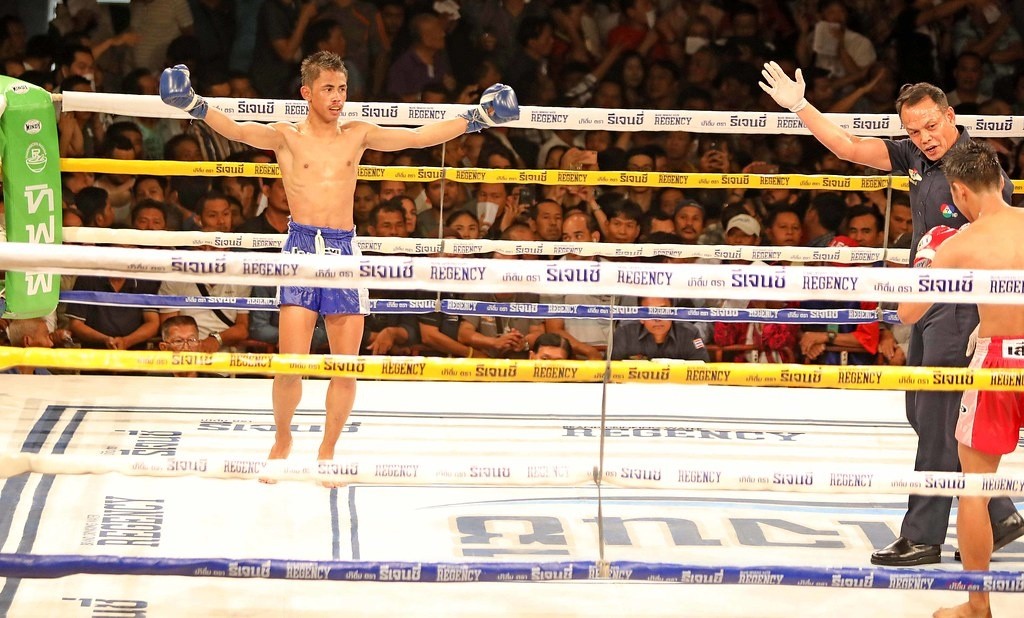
[828,329,837,347]
[209,330,223,349]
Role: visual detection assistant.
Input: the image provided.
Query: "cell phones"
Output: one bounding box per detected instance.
[518,189,531,213]
[709,141,722,162]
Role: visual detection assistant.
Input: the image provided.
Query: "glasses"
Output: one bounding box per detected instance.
[630,166,652,172]
[167,335,199,347]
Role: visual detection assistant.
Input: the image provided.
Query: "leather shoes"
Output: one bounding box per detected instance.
[870,536,942,566]
[955,512,1023,561]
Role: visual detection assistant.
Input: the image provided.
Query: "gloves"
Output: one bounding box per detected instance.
[912,225,956,269]
[966,323,979,358]
[159,64,208,124]
[460,83,520,134]
[759,60,808,114]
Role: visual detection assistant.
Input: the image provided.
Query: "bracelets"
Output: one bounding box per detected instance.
[467,346,474,358]
[591,204,601,211]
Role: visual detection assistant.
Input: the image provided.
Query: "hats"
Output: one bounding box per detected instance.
[726,214,761,238]
[673,199,703,216]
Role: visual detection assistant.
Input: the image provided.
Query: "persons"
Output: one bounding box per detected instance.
[897,142,1024,618]
[0,0,1024,379]
[758,61,1024,566]
[158,49,522,488]
